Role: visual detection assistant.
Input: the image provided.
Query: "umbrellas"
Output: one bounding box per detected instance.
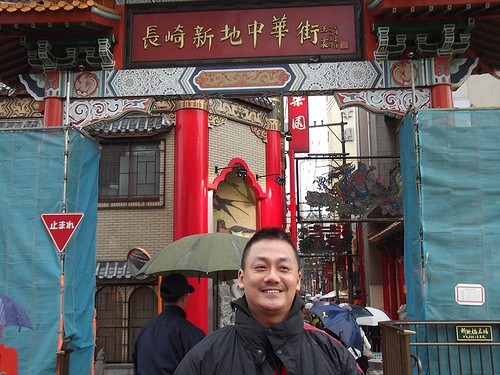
[309,300,339,314]
[312,305,362,352]
[0,293,34,332]
[337,302,373,318]
[133,233,252,329]
[302,290,350,309]
[355,307,391,326]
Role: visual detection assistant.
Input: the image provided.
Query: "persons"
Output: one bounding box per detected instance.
[132,274,206,375]
[312,314,320,326]
[370,326,381,352]
[0,324,17,375]
[315,317,324,330]
[173,227,364,375]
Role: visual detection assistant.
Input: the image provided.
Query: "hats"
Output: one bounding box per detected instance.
[160,273,195,295]
